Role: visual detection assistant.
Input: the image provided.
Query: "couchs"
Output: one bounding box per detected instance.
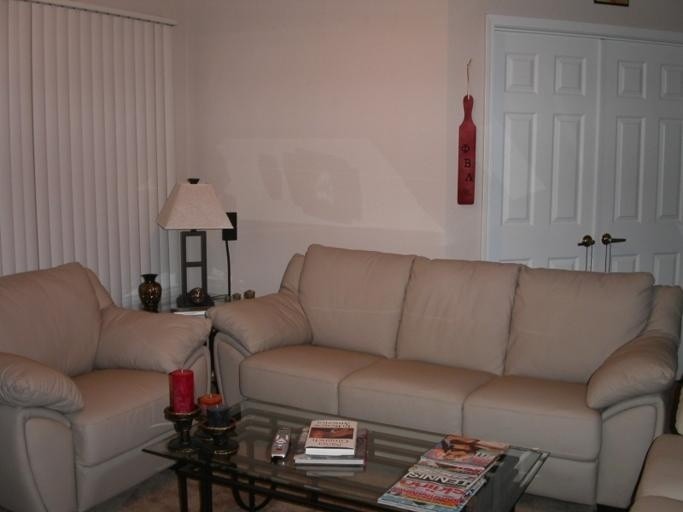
[628,389,680,510]
[202,242,681,510]
[0,260,213,511]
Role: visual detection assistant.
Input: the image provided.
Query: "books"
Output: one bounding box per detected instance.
[172,306,211,316]
[377,434,510,512]
[293,419,369,478]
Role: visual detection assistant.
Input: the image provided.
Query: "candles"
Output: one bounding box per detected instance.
[198,394,222,415]
[168,368,196,412]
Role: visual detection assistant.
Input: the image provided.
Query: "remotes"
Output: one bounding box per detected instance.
[271,424,291,459]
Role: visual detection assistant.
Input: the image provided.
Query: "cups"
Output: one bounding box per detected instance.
[231,293,241,301]
[242,289,255,299]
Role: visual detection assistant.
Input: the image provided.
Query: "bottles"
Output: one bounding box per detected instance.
[138,273,161,311]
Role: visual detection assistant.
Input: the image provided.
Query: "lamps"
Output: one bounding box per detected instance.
[153,177,234,311]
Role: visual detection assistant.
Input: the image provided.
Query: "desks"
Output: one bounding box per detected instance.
[120,288,254,317]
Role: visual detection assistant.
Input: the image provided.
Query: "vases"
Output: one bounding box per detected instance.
[137,273,162,308]
